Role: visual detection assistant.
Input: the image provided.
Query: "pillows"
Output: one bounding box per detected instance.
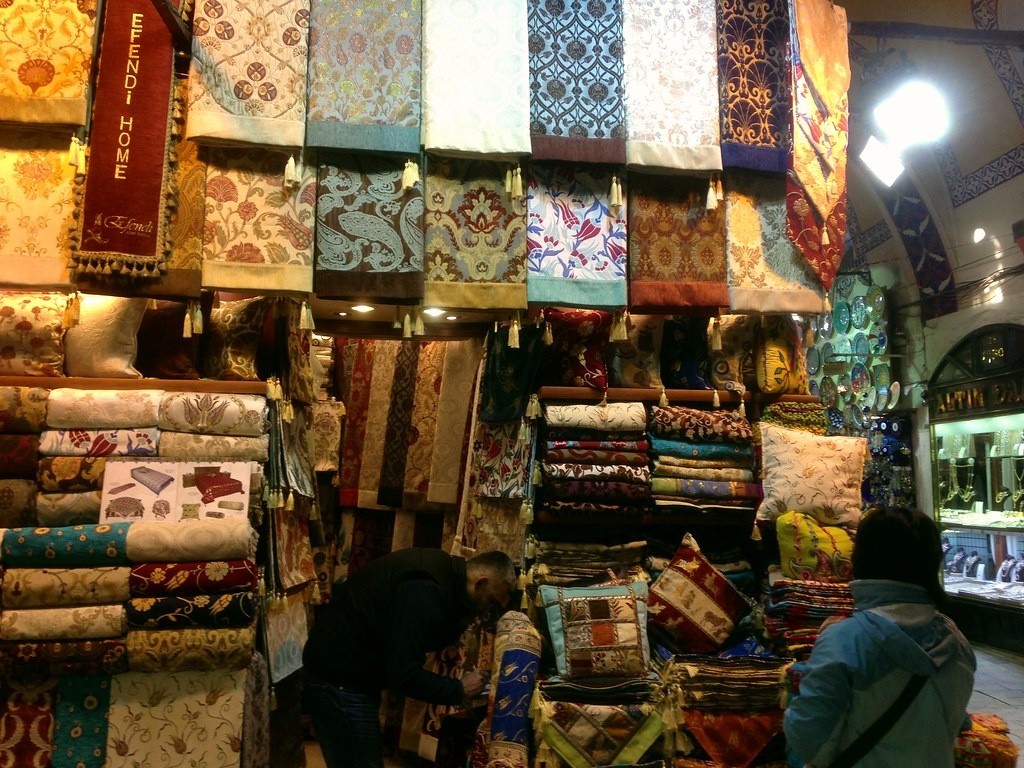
[756,321,812,394]
[776,510,855,581]
[608,314,670,408]
[659,317,720,407]
[753,421,869,530]
[642,532,754,657]
[62,294,150,379]
[202,294,274,381]
[0,286,84,378]
[136,301,200,381]
[536,305,614,406]
[540,581,650,680]
[708,315,755,416]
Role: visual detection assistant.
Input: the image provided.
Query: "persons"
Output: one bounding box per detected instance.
[302,545,518,768]
[784,504,977,767]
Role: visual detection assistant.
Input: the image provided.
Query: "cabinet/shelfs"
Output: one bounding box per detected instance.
[927,322,1024,657]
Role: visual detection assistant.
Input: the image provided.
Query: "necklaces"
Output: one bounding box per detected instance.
[936,428,1023,604]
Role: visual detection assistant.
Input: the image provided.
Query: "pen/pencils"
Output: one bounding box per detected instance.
[469,660,477,672]
